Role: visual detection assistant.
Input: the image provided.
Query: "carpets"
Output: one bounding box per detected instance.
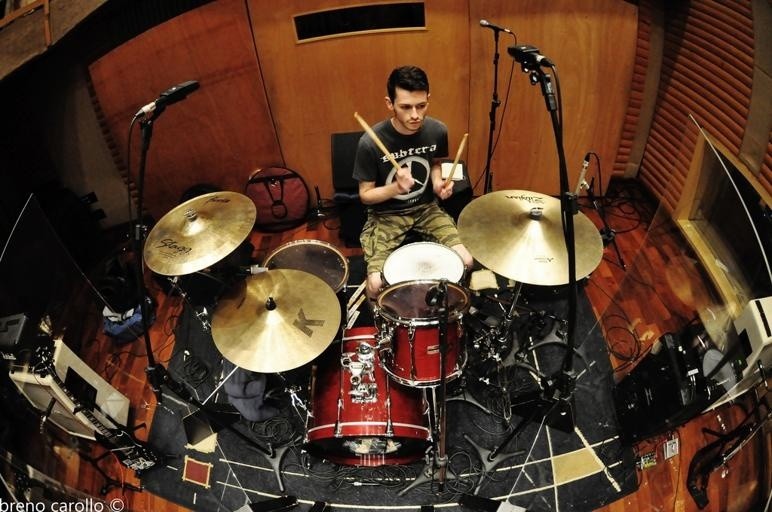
[139,252,643,512]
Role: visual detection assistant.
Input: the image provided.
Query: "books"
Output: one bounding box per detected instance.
[442,162,463,181]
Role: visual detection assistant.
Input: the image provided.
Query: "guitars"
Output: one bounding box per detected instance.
[36,344,161,474]
[697,412,771,478]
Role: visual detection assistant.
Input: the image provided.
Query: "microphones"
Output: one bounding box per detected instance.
[131,79,203,119]
[479,17,512,35]
[506,43,555,74]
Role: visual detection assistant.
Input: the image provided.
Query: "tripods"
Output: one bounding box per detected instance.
[128,122,592,498]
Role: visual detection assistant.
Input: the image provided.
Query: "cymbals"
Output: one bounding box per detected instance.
[457,189,603,286]
[143,190,257,276]
[211,269,341,374]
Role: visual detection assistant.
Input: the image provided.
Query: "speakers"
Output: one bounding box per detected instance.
[613,321,722,447]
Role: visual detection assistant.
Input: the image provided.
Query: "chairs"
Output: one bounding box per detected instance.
[311,129,376,246]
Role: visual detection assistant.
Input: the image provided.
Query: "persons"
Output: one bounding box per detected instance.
[353,66,474,315]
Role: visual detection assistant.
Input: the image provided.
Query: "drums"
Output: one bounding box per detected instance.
[379,241,468,292]
[261,238,350,344]
[373,278,469,389]
[302,326,434,468]
